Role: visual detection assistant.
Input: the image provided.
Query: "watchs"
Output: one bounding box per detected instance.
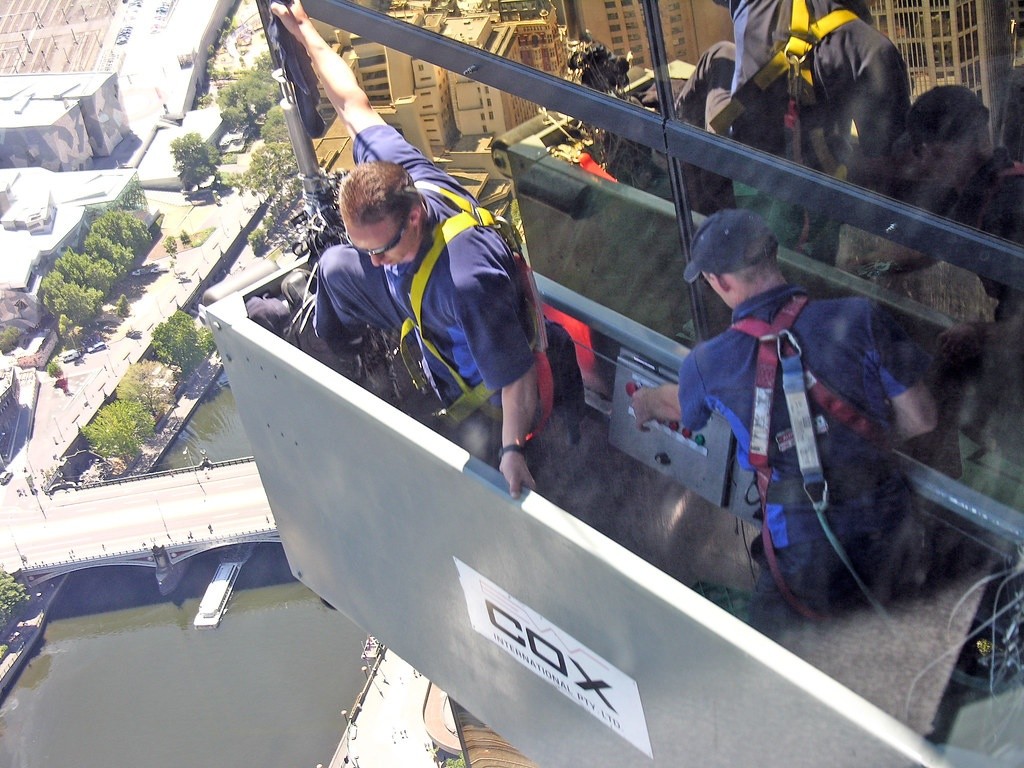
[497,443,528,462]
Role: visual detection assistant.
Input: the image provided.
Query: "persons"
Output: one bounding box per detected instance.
[271,0,547,501]
[633,206,938,634]
[889,89,1023,359]
[668,1,912,233]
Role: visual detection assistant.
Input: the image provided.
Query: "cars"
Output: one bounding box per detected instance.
[63,351,81,364]
[151,267,159,274]
[128,332,141,340]
[131,270,141,276]
[0,472,13,484]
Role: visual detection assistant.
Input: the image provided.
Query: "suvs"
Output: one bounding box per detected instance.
[88,342,106,354]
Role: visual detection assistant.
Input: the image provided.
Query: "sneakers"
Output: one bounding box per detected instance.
[282,266,367,360]
[645,77,696,169]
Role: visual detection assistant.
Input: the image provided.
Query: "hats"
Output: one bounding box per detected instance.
[683,207,779,284]
[910,84,989,156]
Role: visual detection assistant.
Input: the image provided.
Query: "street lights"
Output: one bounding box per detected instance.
[183,446,200,484]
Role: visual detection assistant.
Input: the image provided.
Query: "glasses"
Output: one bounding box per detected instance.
[346,216,406,257]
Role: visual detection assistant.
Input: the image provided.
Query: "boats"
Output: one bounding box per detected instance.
[194,562,240,628]
